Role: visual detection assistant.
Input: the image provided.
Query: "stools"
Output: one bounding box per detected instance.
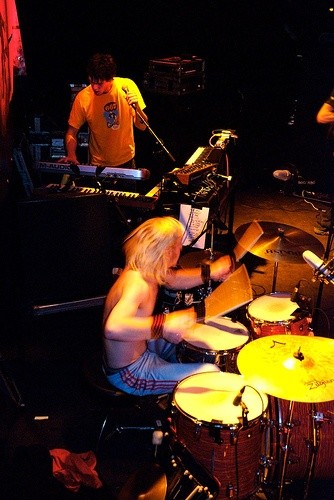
[88,373,171,463]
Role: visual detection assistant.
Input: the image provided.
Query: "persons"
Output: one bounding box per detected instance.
[313,91,334,234]
[56,53,149,170]
[104,217,232,396]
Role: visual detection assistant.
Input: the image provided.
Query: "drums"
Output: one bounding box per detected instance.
[176,315,251,375]
[245,291,313,341]
[267,394,334,500]
[166,370,271,500]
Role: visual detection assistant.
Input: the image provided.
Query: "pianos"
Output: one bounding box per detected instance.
[32,182,159,208]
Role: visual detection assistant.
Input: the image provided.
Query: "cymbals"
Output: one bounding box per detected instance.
[236,334,334,404]
[178,250,243,271]
[234,221,325,265]
[116,463,168,500]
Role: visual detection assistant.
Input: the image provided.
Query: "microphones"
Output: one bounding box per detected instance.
[291,282,300,302]
[233,386,246,407]
[273,170,303,181]
[122,85,136,109]
[302,250,334,285]
